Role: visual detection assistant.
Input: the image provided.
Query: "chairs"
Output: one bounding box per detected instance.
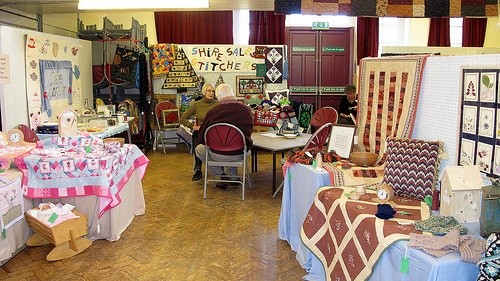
[272,107,338,198]
[156,100,179,154]
[204,123,252,200]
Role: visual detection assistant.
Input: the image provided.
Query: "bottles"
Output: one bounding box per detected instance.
[316,153,322,171]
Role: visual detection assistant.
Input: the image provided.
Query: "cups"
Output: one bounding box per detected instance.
[118,116,124,123]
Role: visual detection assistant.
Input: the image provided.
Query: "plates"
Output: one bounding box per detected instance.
[285,134,296,138]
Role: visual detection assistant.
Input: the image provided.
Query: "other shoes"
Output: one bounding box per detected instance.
[192,172,202,181]
[217,177,240,187]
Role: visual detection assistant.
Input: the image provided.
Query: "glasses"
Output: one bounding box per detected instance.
[206,90,214,93]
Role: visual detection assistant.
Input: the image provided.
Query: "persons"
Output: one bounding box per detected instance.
[180,83,253,188]
[338,84,359,125]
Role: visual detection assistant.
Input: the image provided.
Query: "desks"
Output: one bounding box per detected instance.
[251,131,311,195]
[0,117,141,266]
[283,162,500,281]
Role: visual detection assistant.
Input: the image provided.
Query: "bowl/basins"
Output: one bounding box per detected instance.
[350,152,378,166]
[103,138,125,147]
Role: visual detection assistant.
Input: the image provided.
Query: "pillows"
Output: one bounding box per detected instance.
[383,136,441,203]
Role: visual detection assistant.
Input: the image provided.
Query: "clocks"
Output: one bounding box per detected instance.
[377,185,395,201]
[7,129,24,146]
